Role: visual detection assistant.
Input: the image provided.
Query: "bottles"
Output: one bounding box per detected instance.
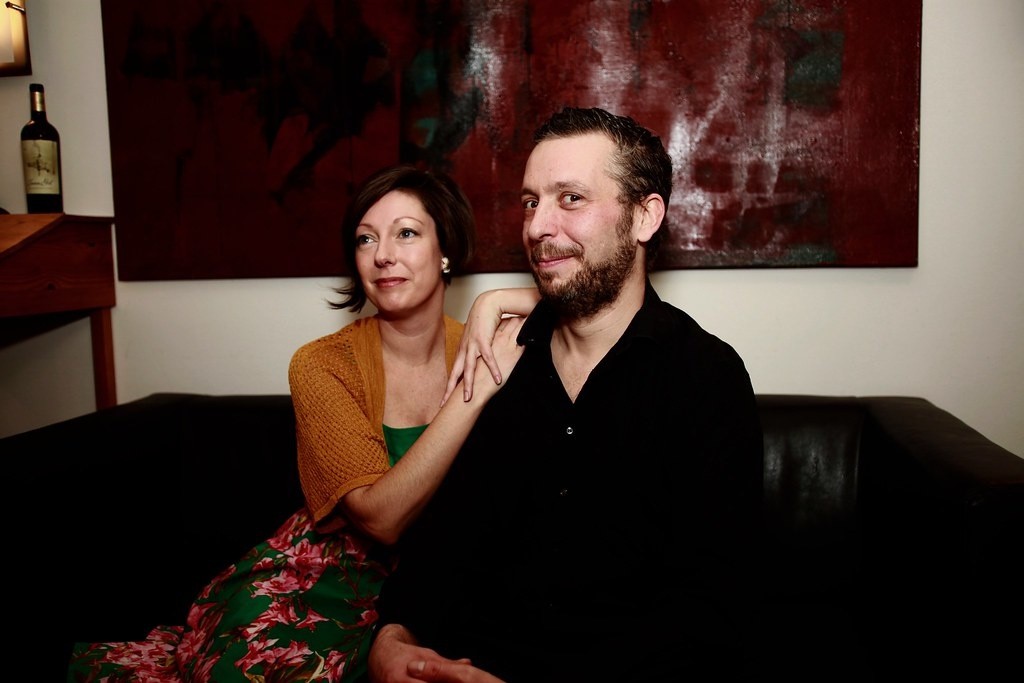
[20,84,63,215]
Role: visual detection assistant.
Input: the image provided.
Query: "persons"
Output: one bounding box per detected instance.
[369,106,760,683]
[63,160,542,682]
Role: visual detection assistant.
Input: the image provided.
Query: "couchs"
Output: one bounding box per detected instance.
[0,390,1024,683]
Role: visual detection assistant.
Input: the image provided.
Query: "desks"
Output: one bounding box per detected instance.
[0,213,119,413]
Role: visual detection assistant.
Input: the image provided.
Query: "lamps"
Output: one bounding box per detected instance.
[0,0,32,77]
